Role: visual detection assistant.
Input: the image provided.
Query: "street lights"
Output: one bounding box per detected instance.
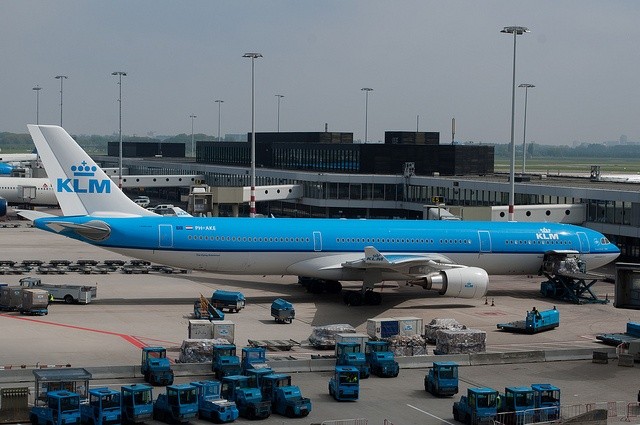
[501,27,532,224]
[361,88,374,144]
[243,54,264,217]
[275,95,285,133]
[215,101,225,142]
[33,85,43,126]
[191,116,197,158]
[112,72,128,192]
[54,76,69,129]
[519,85,536,176]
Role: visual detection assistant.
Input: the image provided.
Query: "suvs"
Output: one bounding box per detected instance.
[147,204,174,212]
[135,197,151,208]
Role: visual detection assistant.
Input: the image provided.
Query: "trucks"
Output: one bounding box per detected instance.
[193,293,224,322]
[28,346,311,425]
[498,388,537,424]
[211,290,246,313]
[424,362,459,398]
[453,387,504,425]
[366,341,399,377]
[271,298,295,324]
[19,278,98,306]
[532,386,561,424]
[329,365,359,401]
[334,340,369,379]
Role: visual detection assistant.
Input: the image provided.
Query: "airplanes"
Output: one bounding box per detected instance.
[1,178,62,218]
[0,154,38,175]
[15,124,622,306]
